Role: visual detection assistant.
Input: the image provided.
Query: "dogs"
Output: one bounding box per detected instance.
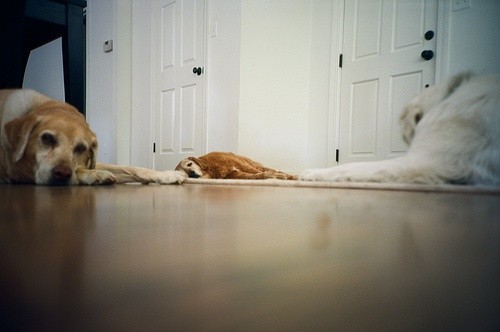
[295,71,498,189]
[174,151,296,179]
[0,89,187,187]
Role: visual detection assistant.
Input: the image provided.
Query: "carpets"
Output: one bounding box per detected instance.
[140,172,500,196]
